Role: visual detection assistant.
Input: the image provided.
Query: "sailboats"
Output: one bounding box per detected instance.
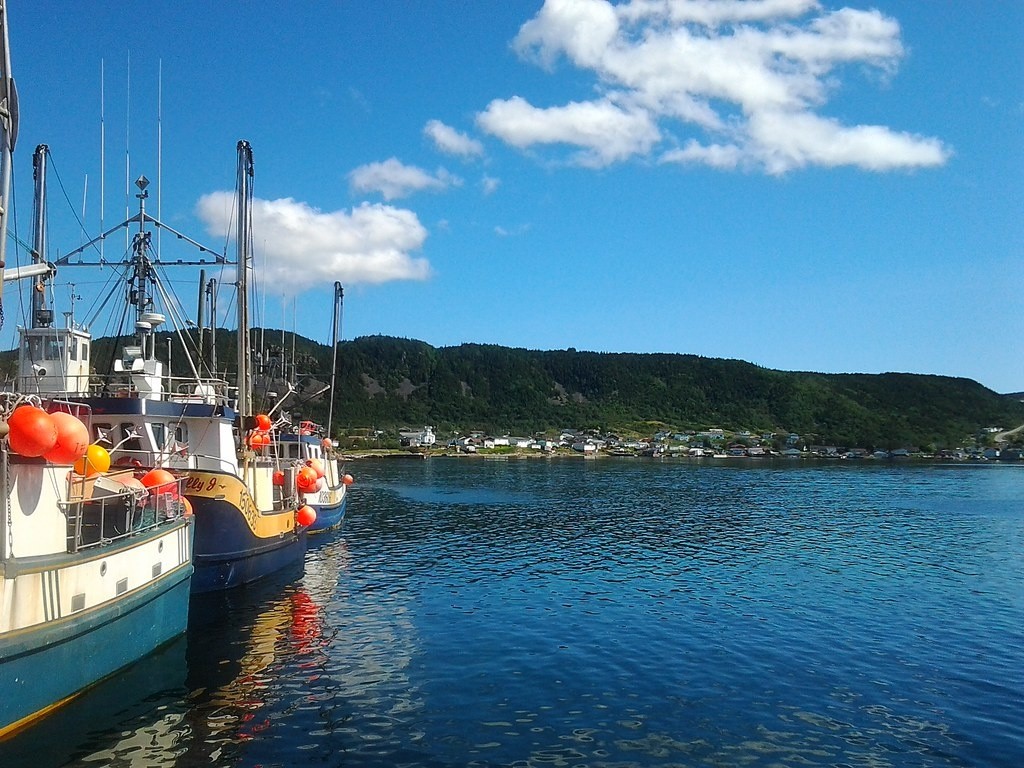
[1,2,354,746]
